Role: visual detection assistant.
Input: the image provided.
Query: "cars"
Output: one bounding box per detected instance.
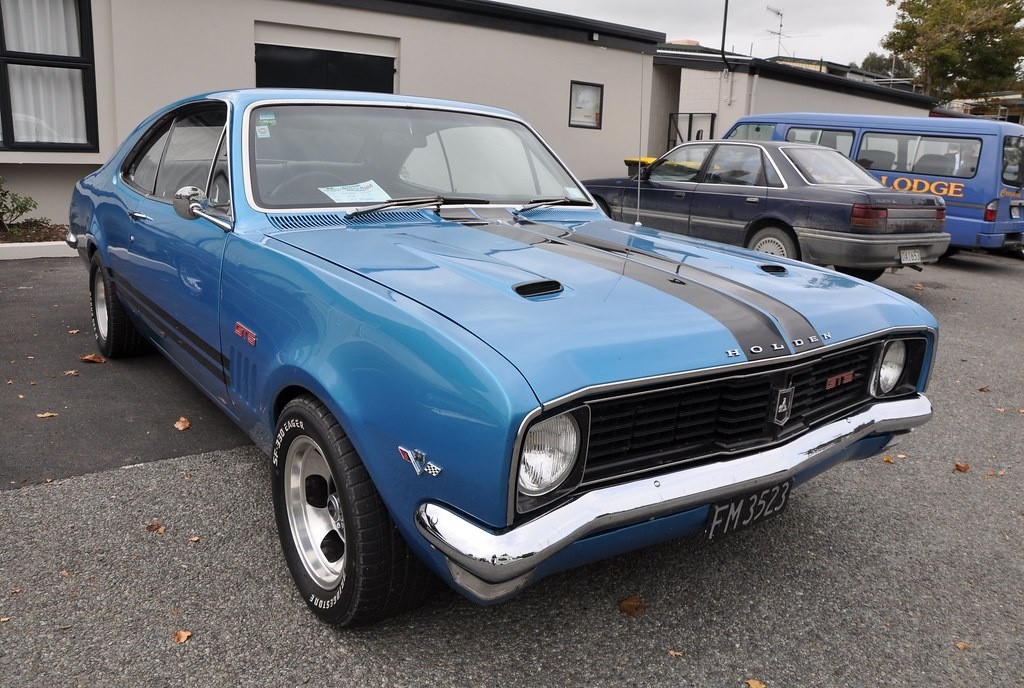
[578,138,953,284]
[63,86,941,629]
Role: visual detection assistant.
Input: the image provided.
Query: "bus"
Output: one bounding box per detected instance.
[708,113,1024,259]
